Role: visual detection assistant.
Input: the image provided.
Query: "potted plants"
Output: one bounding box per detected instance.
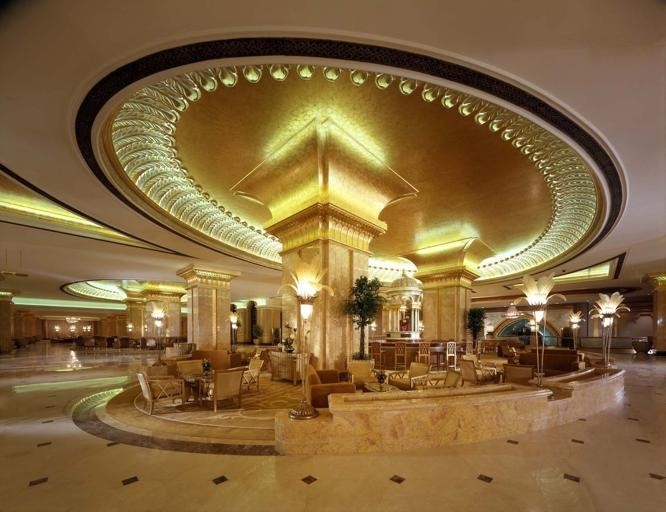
[252,325,263,345]
[343,275,387,386]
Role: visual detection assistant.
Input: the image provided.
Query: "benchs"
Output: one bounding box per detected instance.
[502,363,536,384]
[518,347,586,376]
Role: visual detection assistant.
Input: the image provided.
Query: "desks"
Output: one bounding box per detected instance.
[268,351,312,385]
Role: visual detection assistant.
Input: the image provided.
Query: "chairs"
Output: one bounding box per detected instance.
[80,335,130,349]
[437,341,457,371]
[414,343,431,364]
[136,342,265,414]
[388,362,432,391]
[459,359,499,387]
[413,369,461,390]
[370,341,386,370]
[304,364,356,408]
[460,354,497,376]
[394,343,407,371]
